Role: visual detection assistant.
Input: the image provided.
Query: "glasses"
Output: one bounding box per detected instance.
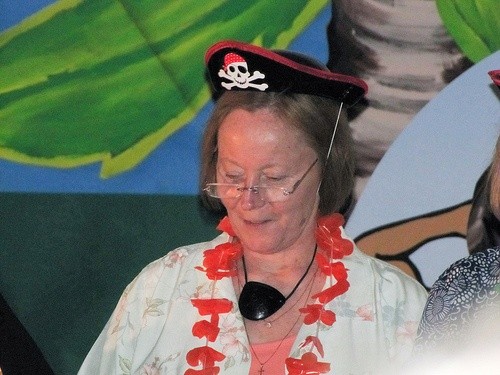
[204,156,319,202]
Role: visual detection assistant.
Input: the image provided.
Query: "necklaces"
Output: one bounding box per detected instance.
[186,236,353,375]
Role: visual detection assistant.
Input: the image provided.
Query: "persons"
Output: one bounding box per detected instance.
[77,40,431,375]
[412,137,500,374]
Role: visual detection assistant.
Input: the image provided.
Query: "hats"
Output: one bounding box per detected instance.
[204,39,369,108]
